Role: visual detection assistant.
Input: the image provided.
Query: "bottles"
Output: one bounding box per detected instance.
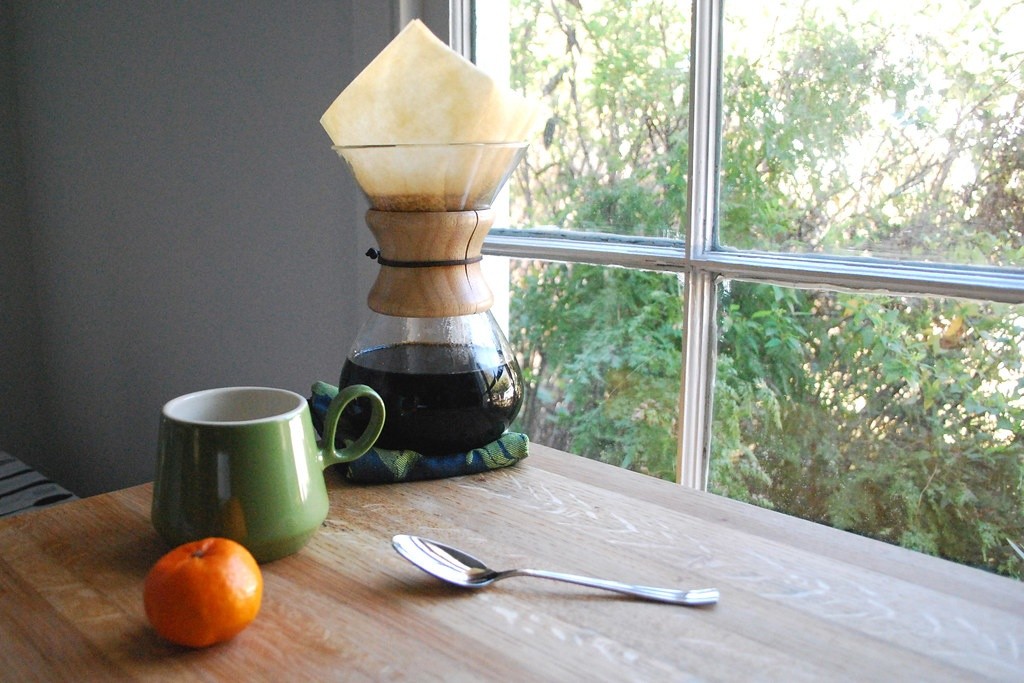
[313,212,534,462]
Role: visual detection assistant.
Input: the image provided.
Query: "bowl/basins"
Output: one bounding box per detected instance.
[329,140,531,211]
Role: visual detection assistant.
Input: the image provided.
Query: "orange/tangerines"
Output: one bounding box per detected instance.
[143,537,264,648]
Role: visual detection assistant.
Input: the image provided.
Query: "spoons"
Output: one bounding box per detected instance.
[390,534,718,618]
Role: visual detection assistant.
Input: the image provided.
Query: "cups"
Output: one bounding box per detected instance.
[151,384,385,564]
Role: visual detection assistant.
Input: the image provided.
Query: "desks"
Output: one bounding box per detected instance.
[0,423,1024,683]
